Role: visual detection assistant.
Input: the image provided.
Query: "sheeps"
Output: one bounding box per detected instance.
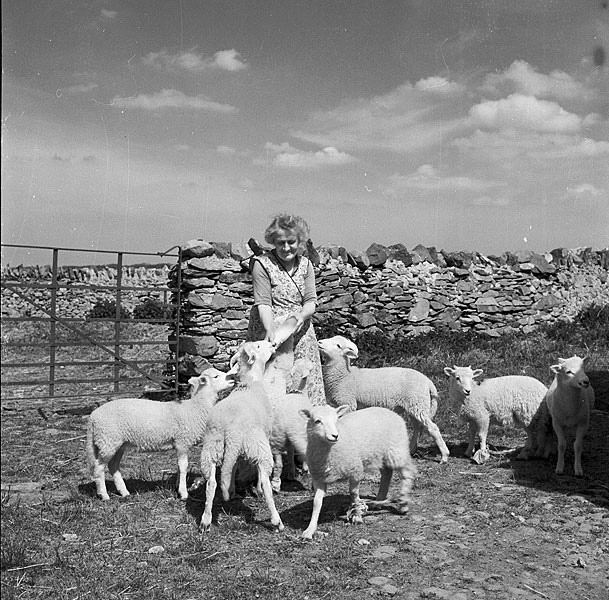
[297,405,417,542]
[443,365,551,458]
[198,340,285,541]
[86,368,236,502]
[317,335,450,465]
[269,359,327,500]
[546,356,595,476]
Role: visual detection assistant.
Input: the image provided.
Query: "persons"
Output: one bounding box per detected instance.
[244,212,329,475]
